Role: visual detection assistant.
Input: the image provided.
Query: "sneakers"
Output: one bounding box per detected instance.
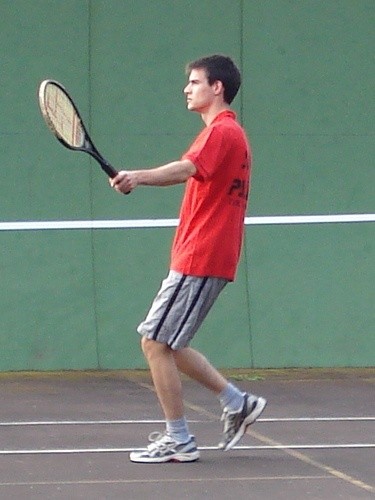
[129,434,200,463]
[218,392,268,452]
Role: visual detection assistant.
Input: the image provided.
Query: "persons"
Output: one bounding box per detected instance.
[109,55,269,463]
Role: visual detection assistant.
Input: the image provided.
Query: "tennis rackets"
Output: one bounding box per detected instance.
[38,79,131,195]
